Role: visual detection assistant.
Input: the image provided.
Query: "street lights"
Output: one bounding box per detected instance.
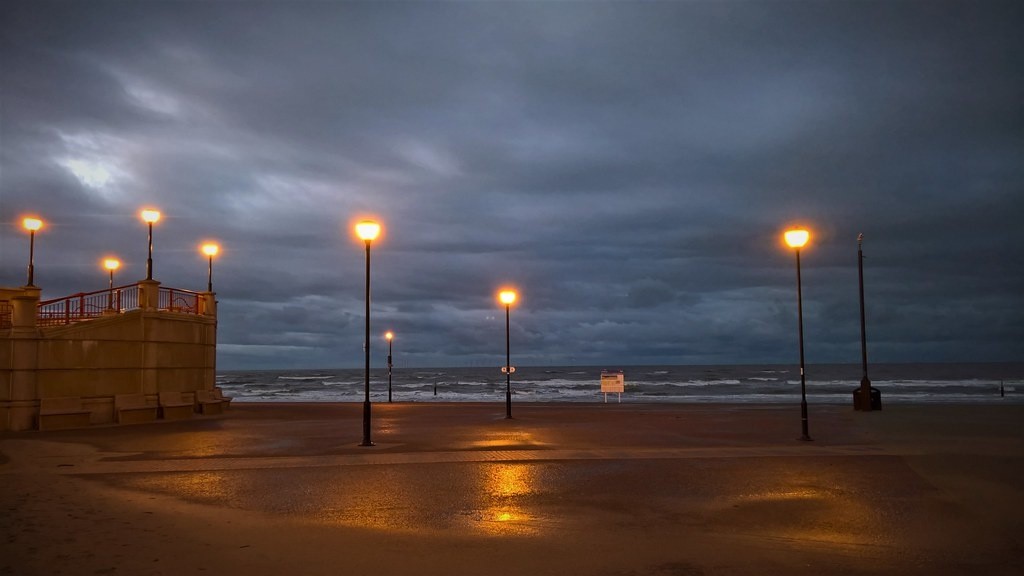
[499,290,518,420]
[385,332,393,403]
[141,208,162,279]
[784,228,816,441]
[354,219,383,446]
[103,257,122,309]
[21,217,44,286]
[201,242,220,292]
